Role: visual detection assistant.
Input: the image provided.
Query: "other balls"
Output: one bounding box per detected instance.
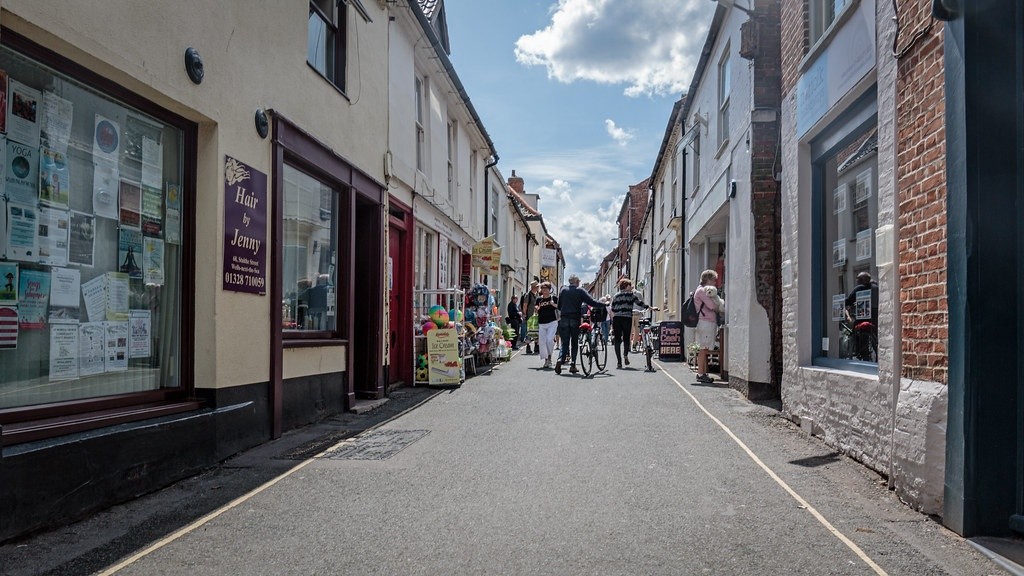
[416,305,463,336]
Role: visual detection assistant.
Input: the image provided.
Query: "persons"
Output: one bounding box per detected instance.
[121,246,141,277]
[628,286,644,352]
[507,274,611,375]
[40,171,50,200]
[51,174,60,201]
[693,270,720,384]
[5,272,14,294]
[841,272,878,363]
[611,279,658,368]
[535,282,558,369]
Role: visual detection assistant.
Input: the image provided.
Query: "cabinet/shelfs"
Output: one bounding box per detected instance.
[412,285,465,387]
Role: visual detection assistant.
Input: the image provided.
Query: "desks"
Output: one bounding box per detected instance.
[688,351,720,372]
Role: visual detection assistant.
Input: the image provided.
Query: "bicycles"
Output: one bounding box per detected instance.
[578,302,611,376]
[632,307,660,372]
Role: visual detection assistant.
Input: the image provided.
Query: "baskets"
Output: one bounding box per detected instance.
[590,307,608,323]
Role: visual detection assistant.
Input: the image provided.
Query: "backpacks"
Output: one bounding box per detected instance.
[681,292,705,328]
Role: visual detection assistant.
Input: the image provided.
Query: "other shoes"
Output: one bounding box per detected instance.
[555,361,561,375]
[696,373,715,383]
[570,363,576,374]
[625,358,629,365]
[534,344,540,354]
[511,341,519,350]
[617,363,622,367]
[543,359,552,368]
[526,345,533,354]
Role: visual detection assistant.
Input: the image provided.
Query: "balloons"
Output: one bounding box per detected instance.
[422,305,463,336]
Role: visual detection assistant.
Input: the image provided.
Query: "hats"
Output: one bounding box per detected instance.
[530,280,540,288]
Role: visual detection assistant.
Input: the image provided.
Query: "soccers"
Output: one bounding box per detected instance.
[416,367,428,381]
[417,353,428,367]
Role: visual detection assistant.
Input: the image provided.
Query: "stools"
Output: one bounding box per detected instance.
[464,354,477,375]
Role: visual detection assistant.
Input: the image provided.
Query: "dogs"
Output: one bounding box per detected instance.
[703,286,725,313]
